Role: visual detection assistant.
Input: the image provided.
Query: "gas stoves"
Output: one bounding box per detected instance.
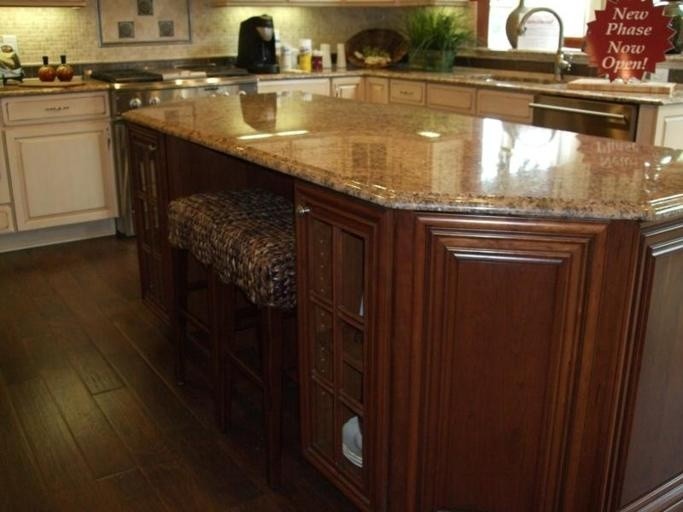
[91,60,249,83]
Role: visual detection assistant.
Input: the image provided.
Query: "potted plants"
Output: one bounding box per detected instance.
[399,3,483,73]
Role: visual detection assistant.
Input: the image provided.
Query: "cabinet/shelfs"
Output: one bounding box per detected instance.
[0,92,118,252]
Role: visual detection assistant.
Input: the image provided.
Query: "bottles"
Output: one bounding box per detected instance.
[280,39,347,72]
[54,54,73,81]
[36,55,56,82]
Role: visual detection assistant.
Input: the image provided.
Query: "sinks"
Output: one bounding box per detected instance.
[454,74,567,86]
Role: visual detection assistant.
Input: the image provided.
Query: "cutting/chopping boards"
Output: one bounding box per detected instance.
[565,75,679,94]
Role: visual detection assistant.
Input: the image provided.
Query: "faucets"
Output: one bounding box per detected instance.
[516,8,564,52]
